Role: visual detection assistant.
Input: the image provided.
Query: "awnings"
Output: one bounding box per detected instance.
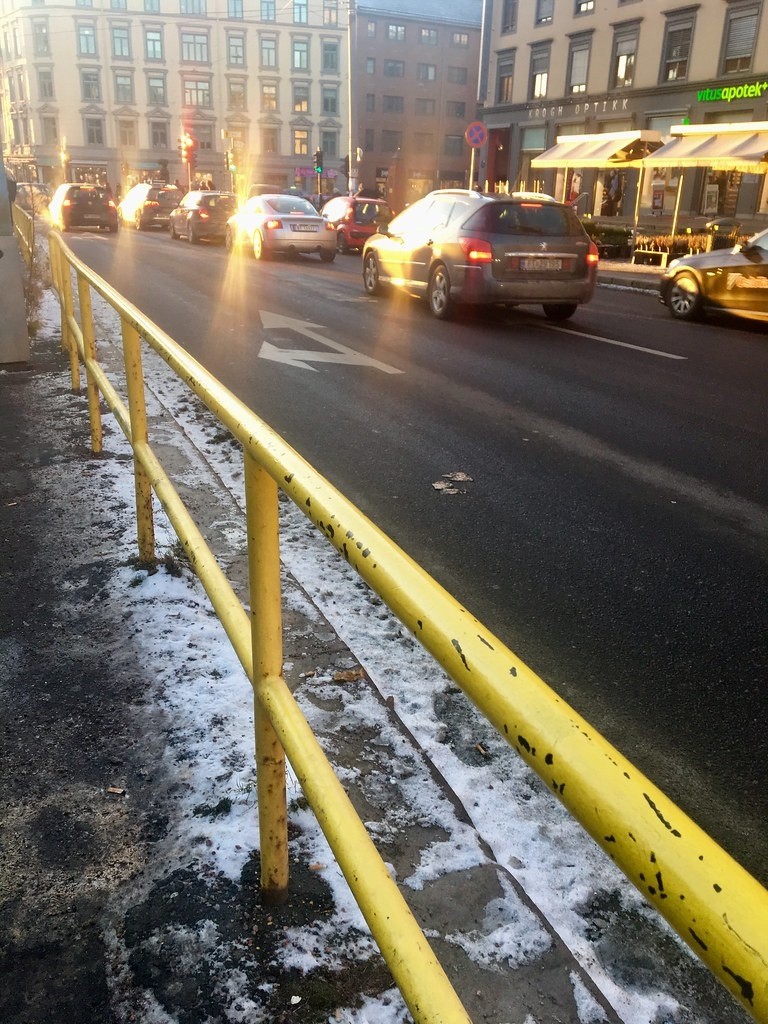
[530,120,768,262]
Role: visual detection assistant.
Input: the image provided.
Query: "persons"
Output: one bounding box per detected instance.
[171,180,216,196]
[355,181,381,199]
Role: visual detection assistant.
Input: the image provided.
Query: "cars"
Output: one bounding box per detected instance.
[320,196,389,253]
[658,223,768,324]
[50,181,118,233]
[223,192,337,264]
[167,190,237,244]
[359,186,598,323]
[117,181,183,230]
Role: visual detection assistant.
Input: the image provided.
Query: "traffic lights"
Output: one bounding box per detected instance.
[178,138,200,171]
[311,149,322,175]
[223,146,241,176]
